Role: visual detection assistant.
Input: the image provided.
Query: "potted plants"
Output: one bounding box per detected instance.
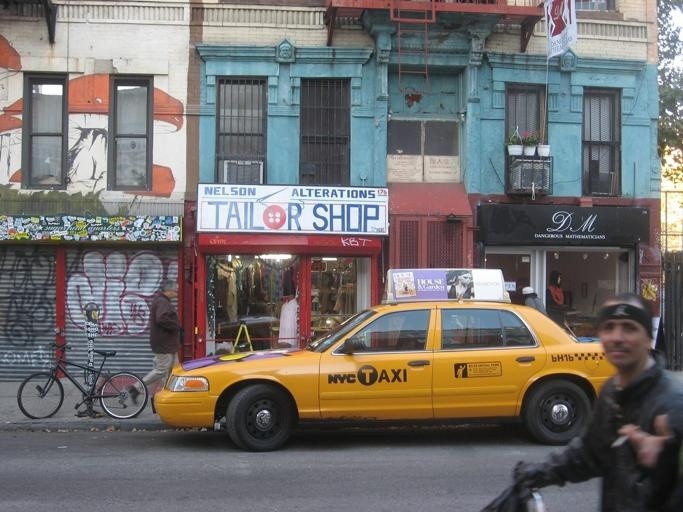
[507,130,523,155]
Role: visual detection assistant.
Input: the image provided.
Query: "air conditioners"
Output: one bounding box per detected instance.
[511,160,550,189]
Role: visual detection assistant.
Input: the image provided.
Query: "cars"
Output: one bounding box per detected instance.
[145,265,617,453]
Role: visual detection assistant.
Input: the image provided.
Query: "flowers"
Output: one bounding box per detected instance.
[523,131,540,146]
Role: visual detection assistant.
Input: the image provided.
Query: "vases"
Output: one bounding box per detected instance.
[524,144,536,156]
[537,145,550,156]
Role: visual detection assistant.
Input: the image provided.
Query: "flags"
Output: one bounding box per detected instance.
[544,0,577,59]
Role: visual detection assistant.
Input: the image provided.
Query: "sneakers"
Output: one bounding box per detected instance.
[126,385,140,405]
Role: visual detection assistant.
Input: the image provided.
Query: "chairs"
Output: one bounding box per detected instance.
[397,321,416,351]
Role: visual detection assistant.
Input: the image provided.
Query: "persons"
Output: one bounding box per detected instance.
[515,297,683,512]
[620,415,682,512]
[127,279,180,414]
[447,270,474,298]
[547,270,564,304]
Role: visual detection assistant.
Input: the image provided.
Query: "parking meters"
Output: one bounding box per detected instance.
[81,302,101,418]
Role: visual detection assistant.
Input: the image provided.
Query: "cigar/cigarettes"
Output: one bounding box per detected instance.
[611,436,628,448]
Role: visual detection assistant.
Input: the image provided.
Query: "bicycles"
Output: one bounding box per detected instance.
[502,460,549,512]
[15,341,149,423]
